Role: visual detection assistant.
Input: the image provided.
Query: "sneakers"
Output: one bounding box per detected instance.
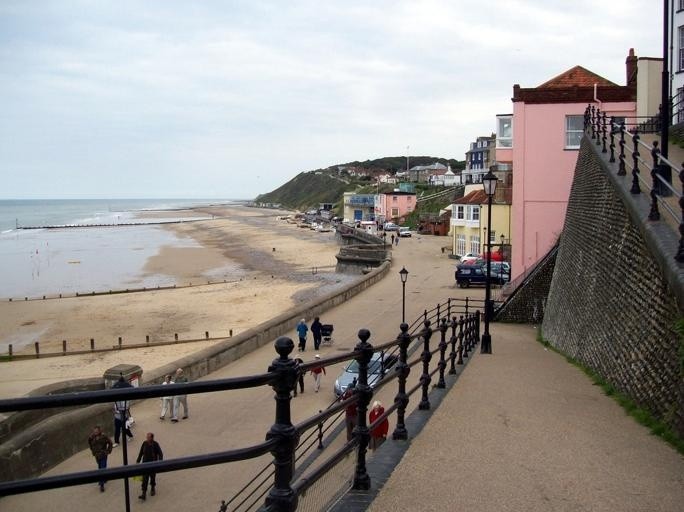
[160,416,188,422]
[101,485,104,491]
[112,443,119,448]
[128,435,133,442]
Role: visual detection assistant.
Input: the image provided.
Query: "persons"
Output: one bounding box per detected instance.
[309,316,324,351]
[134,431,163,501]
[390,234,394,245]
[295,318,308,352]
[169,367,189,422]
[383,231,387,240]
[367,399,389,452]
[397,230,400,240]
[341,382,358,443]
[110,400,134,447]
[292,353,305,398]
[308,353,326,393]
[157,374,175,420]
[380,233,383,239]
[394,236,399,247]
[86,425,112,494]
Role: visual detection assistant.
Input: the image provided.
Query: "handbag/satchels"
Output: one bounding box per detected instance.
[133,475,143,481]
[125,416,135,429]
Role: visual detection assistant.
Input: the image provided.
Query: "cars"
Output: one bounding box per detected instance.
[396,226,413,238]
[333,348,399,403]
[454,247,509,289]
[383,222,400,232]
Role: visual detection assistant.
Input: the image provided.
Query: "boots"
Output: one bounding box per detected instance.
[138,487,155,500]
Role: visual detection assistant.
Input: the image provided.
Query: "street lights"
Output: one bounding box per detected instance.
[479,169,500,354]
[108,372,139,511]
[399,265,408,328]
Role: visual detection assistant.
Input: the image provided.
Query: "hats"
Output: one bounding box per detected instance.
[315,355,320,359]
[348,383,355,390]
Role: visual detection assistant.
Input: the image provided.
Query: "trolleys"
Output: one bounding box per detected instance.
[321,323,334,346]
